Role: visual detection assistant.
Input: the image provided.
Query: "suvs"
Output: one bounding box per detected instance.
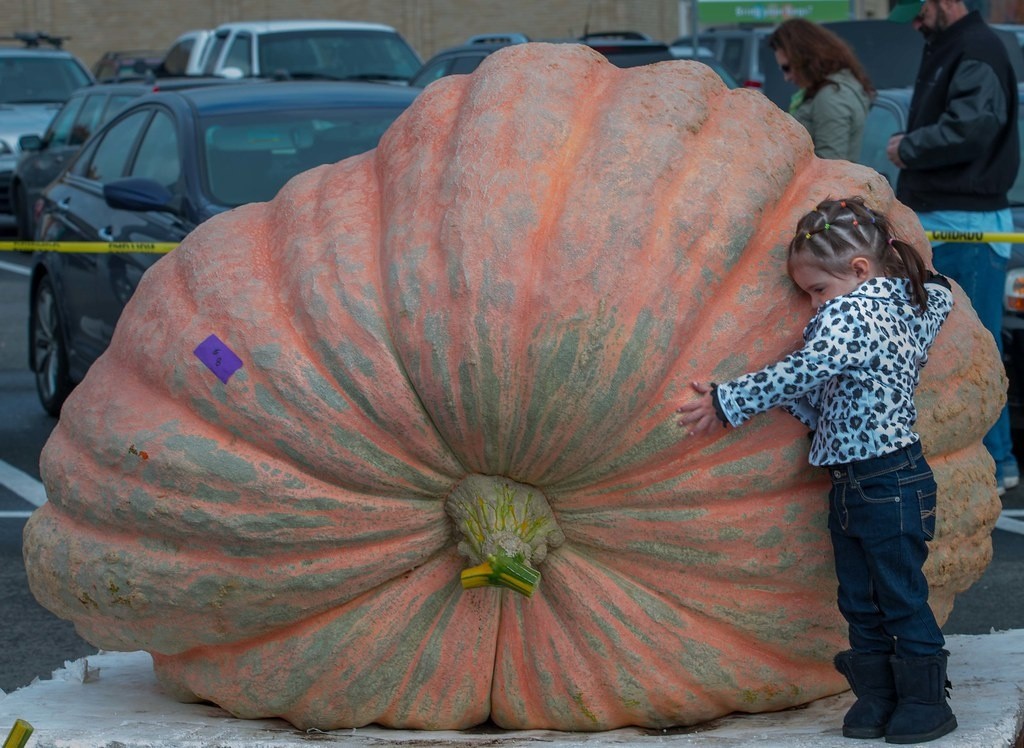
[670,19,778,93]
[314,30,676,148]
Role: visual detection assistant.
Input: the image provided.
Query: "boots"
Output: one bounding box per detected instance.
[832,648,959,745]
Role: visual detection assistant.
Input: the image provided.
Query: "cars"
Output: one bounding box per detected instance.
[88,49,162,79]
[10,83,234,252]
[0,33,97,217]
[852,88,1024,408]
[26,80,424,416]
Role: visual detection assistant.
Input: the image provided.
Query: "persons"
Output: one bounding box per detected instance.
[766,17,875,163]
[676,195,959,744]
[885,0,1022,497]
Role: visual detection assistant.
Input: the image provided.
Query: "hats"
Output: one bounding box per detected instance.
[886,0,926,26]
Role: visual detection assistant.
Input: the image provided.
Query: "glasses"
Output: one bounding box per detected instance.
[777,57,795,74]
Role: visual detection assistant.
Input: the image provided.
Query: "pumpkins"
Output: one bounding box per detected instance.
[22,41,1010,730]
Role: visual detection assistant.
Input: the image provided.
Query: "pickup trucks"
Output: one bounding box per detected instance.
[148,21,425,168]
